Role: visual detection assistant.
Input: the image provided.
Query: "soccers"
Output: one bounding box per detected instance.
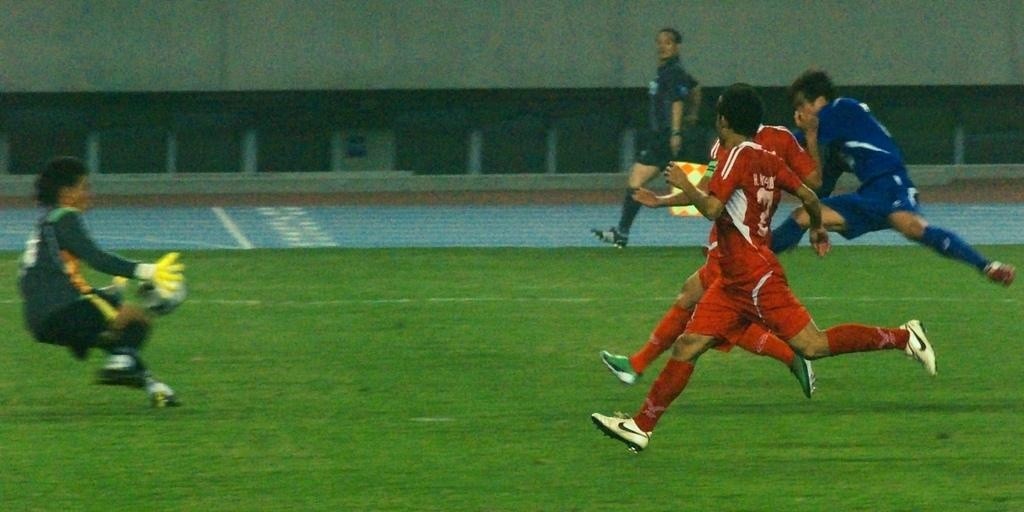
[138,272,186,315]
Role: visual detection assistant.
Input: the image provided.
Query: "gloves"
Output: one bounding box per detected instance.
[147,248,190,297]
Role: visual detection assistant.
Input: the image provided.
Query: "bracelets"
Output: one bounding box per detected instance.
[671,129,681,136]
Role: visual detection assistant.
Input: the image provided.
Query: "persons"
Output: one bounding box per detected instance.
[587,27,704,251]
[594,80,826,401]
[586,81,938,454]
[16,155,190,409]
[762,70,1018,288]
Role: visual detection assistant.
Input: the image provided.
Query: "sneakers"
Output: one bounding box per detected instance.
[590,411,653,453]
[983,259,1016,289]
[145,381,181,410]
[589,225,629,249]
[599,349,639,385]
[701,243,710,258]
[788,353,816,399]
[898,319,938,377]
[98,352,150,388]
